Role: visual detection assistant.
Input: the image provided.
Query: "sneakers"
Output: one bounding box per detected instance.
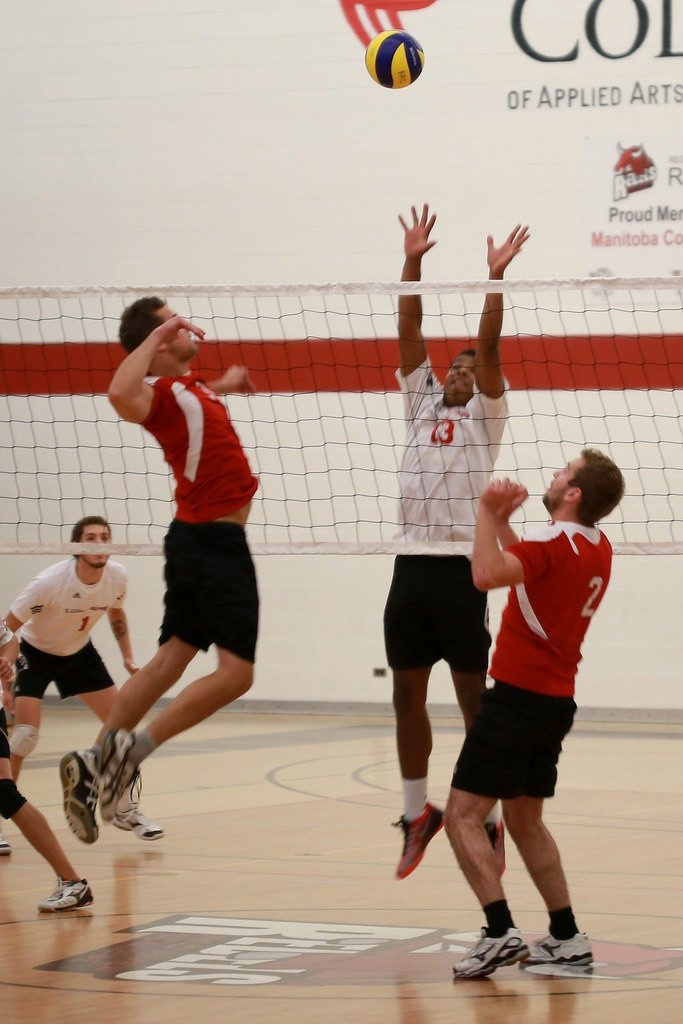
[390,802,444,879]
[38,877,95,913]
[521,932,593,965]
[112,809,165,841]
[484,819,506,880]
[0,840,12,856]
[60,748,104,844]
[452,927,530,978]
[97,728,141,822]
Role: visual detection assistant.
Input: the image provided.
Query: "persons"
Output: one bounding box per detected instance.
[383,202,531,880]
[59,295,260,845]
[0,516,141,855]
[443,449,625,979]
[0,615,94,913]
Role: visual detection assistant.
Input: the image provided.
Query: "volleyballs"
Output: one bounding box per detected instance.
[364,28,425,89]
